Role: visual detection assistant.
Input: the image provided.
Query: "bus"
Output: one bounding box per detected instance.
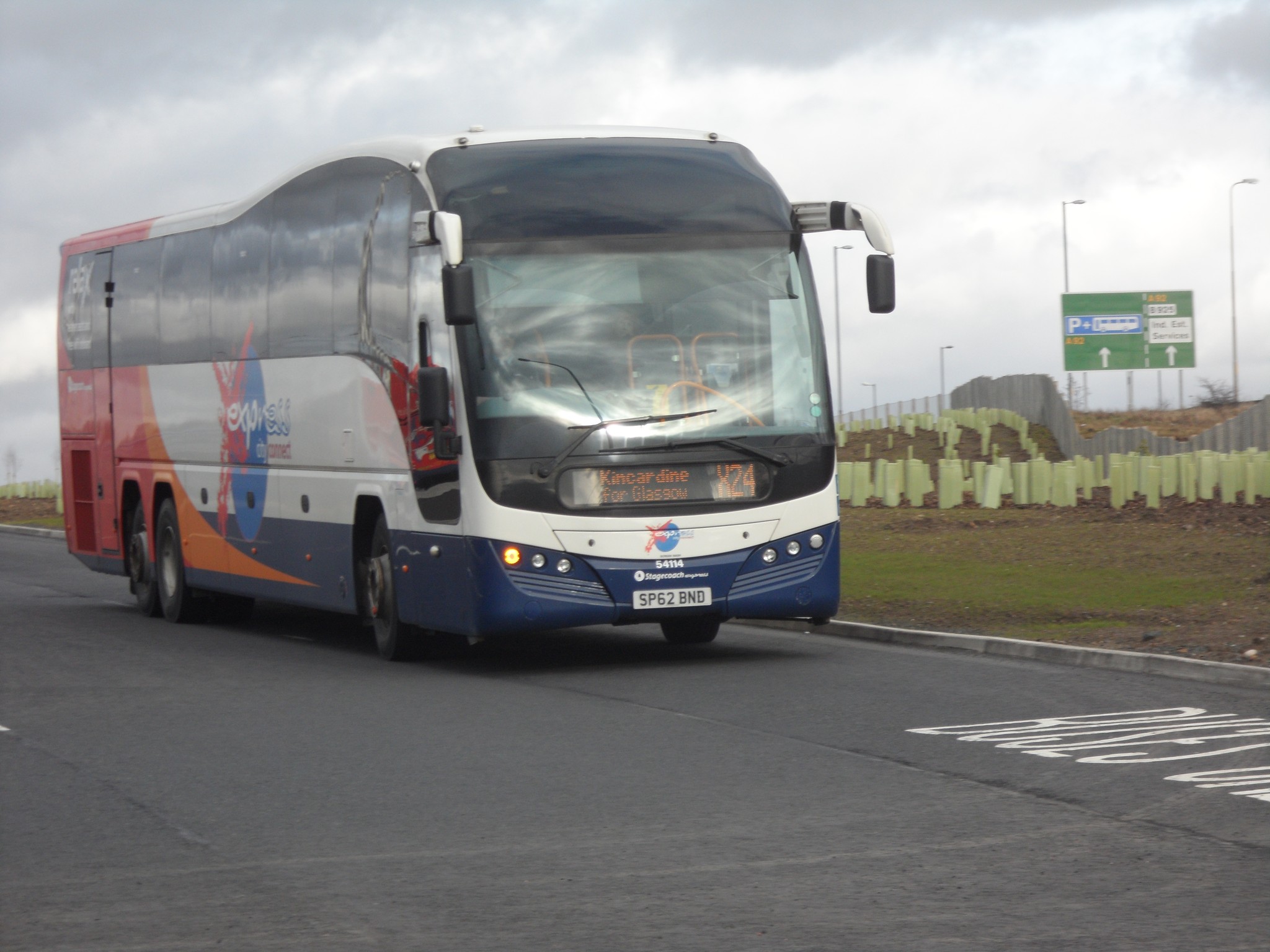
[51,121,900,662]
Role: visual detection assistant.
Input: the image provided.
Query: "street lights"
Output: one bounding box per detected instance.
[940,342,954,414]
[829,240,856,427]
[1060,194,1086,410]
[1226,176,1257,402]
[862,380,878,428]
